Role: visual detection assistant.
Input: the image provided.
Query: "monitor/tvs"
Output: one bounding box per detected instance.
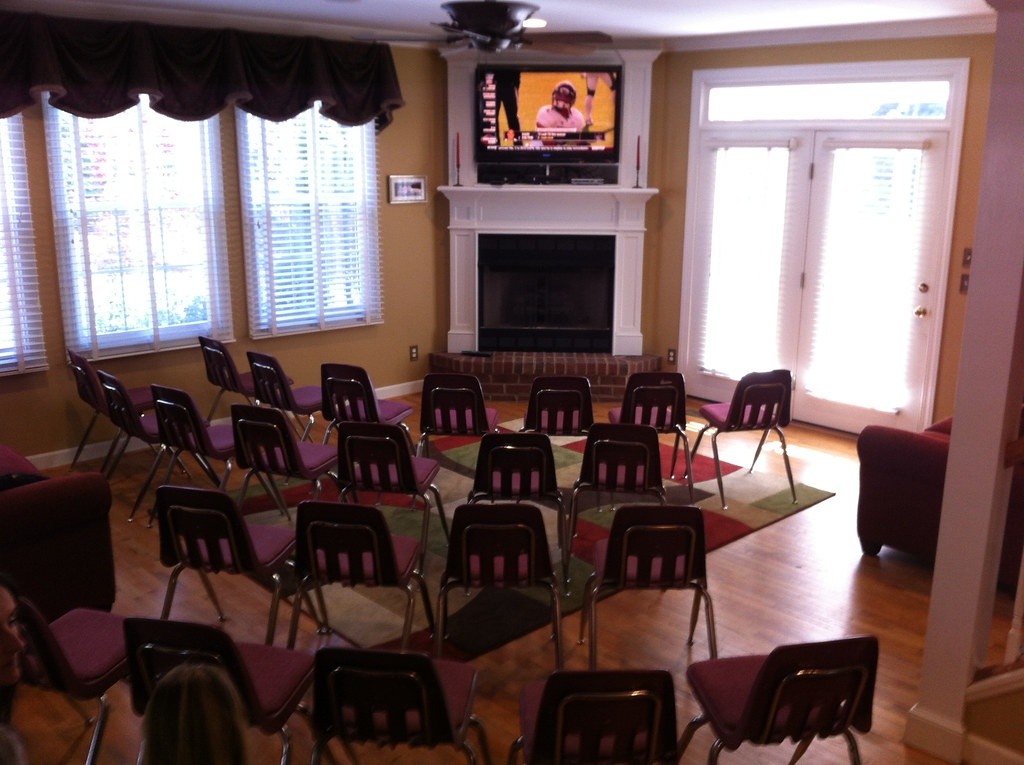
[473,64,622,185]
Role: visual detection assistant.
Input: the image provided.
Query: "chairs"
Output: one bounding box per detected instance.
[16,334,884,765]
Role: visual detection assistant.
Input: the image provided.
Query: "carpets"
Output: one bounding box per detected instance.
[113,415,837,665]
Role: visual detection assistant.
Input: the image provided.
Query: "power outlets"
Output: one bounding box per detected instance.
[410,345,419,362]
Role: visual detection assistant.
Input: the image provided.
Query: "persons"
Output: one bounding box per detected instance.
[579,71,617,126]
[505,129,518,142]
[536,82,587,148]
[489,69,524,148]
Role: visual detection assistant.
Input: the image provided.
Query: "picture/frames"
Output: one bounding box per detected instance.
[387,174,429,204]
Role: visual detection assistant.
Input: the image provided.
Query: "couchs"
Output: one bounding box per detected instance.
[855,412,1024,599]
[0,440,120,622]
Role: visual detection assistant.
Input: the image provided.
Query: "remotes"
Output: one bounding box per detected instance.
[461,350,493,358]
